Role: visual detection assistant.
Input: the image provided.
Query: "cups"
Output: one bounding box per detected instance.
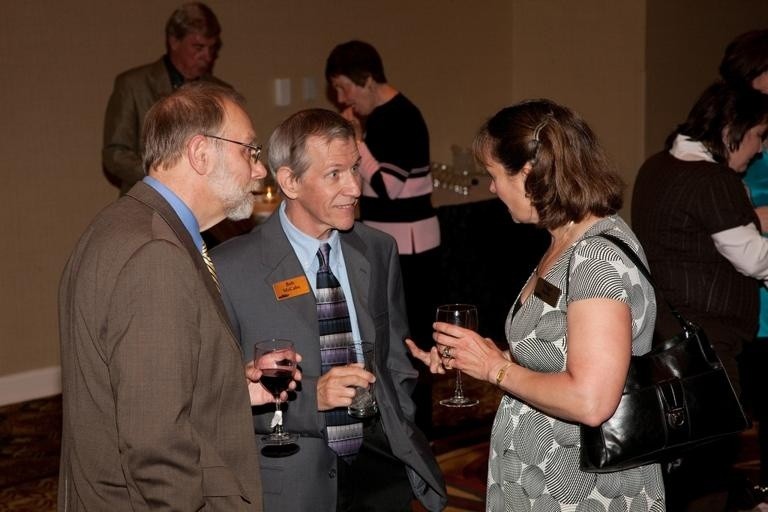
[347,343,378,417]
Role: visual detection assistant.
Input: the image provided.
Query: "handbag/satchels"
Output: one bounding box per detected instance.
[578,319,751,473]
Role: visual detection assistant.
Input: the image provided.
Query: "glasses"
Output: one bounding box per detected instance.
[201,135,262,165]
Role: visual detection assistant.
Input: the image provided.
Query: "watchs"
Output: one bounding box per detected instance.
[496,362,515,387]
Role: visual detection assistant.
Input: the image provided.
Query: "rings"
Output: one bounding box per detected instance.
[445,357,452,370]
[443,346,451,358]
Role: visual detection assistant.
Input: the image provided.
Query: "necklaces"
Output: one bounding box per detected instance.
[536,221,574,276]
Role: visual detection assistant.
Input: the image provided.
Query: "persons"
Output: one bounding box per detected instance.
[720,28,768,500]
[403,97,666,512]
[58,80,304,512]
[207,108,448,512]
[630,79,768,512]
[101,3,224,249]
[325,39,441,447]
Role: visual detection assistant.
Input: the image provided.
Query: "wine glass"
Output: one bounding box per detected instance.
[436,304,480,407]
[252,339,299,445]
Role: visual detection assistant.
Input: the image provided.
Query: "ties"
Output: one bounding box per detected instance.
[316,243,364,467]
[201,238,222,297]
